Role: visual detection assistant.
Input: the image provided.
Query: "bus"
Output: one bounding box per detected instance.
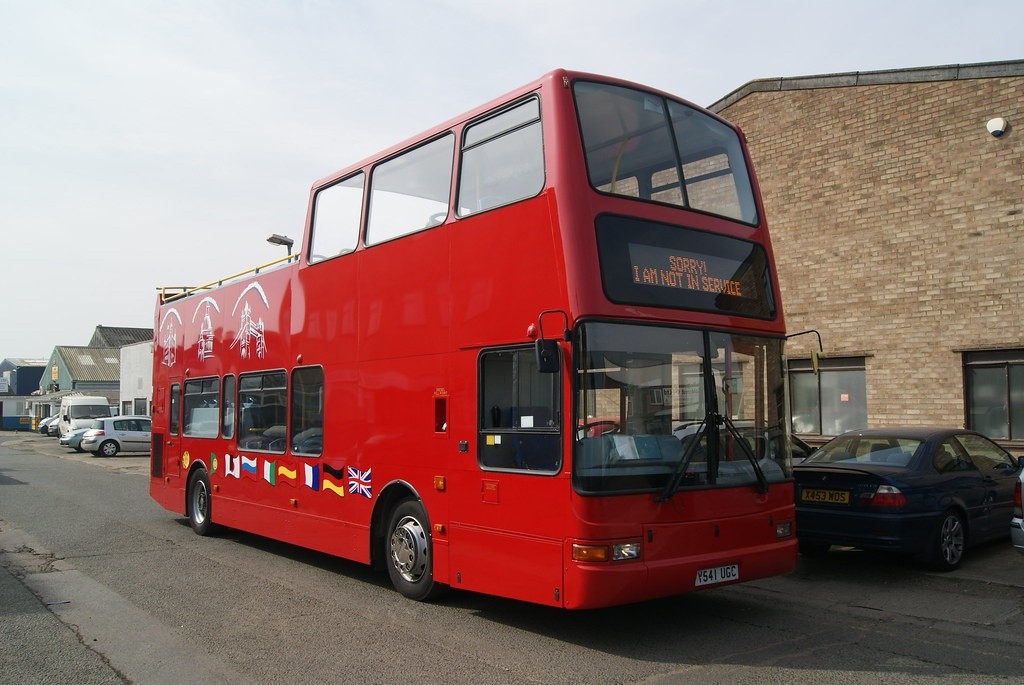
[148,66,823,612]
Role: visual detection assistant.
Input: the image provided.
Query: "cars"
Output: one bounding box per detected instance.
[80,415,153,457]
[37,412,60,435]
[791,425,1024,570]
[58,428,90,452]
[47,417,61,437]
[672,419,820,486]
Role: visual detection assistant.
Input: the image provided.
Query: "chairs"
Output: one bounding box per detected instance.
[887,453,910,464]
[870,442,891,463]
[246,435,323,457]
[509,405,562,471]
[831,452,856,462]
[732,437,753,461]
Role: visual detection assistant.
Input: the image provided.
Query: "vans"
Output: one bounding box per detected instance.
[58,395,112,436]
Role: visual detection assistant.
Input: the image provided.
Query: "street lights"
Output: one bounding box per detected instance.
[266,234,294,261]
